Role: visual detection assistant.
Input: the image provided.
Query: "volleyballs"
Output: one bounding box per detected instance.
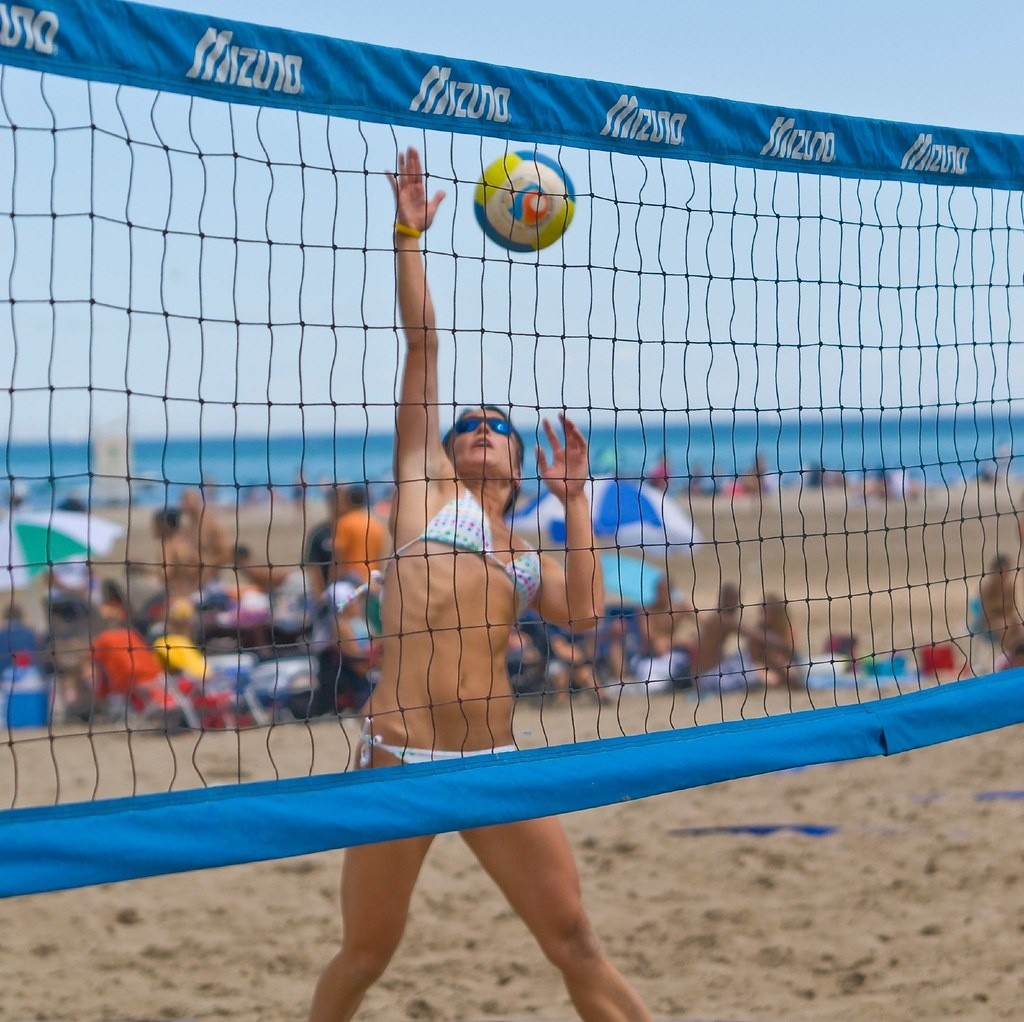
[473,151,575,256]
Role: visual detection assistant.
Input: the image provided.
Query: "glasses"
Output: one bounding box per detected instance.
[455,416,514,435]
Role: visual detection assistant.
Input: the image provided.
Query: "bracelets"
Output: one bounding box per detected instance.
[393,223,422,239]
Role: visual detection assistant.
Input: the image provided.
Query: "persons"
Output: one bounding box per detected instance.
[307,145,657,1022]
[0,433,1024,725]
